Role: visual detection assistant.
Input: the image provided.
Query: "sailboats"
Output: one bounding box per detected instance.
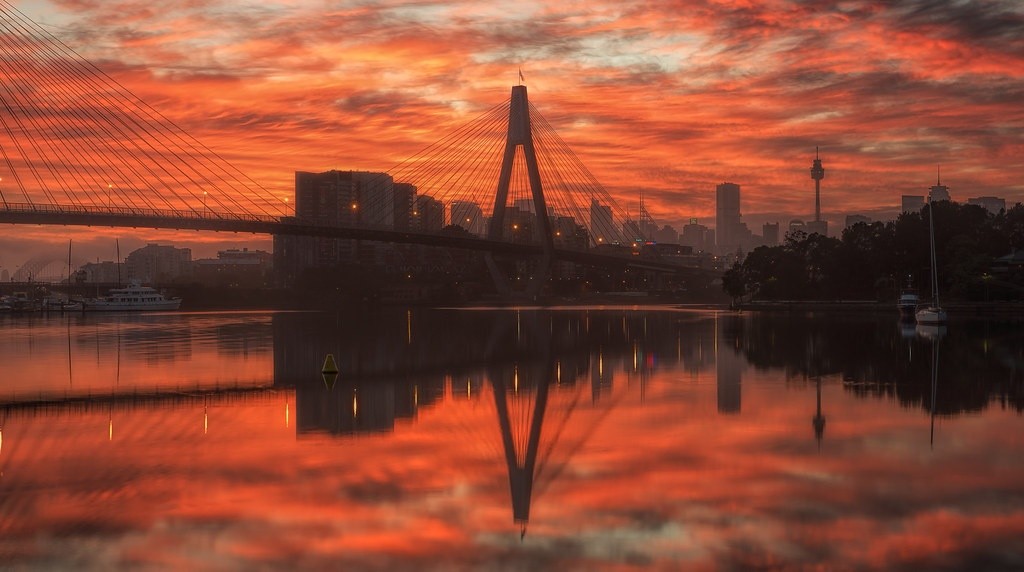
[915,322,947,449]
[915,187,947,326]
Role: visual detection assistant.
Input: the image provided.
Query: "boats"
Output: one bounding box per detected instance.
[895,273,923,308]
[0,237,184,312]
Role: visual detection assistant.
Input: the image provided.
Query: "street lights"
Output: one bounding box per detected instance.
[108,184,111,213]
[203,191,207,219]
[286,197,288,217]
